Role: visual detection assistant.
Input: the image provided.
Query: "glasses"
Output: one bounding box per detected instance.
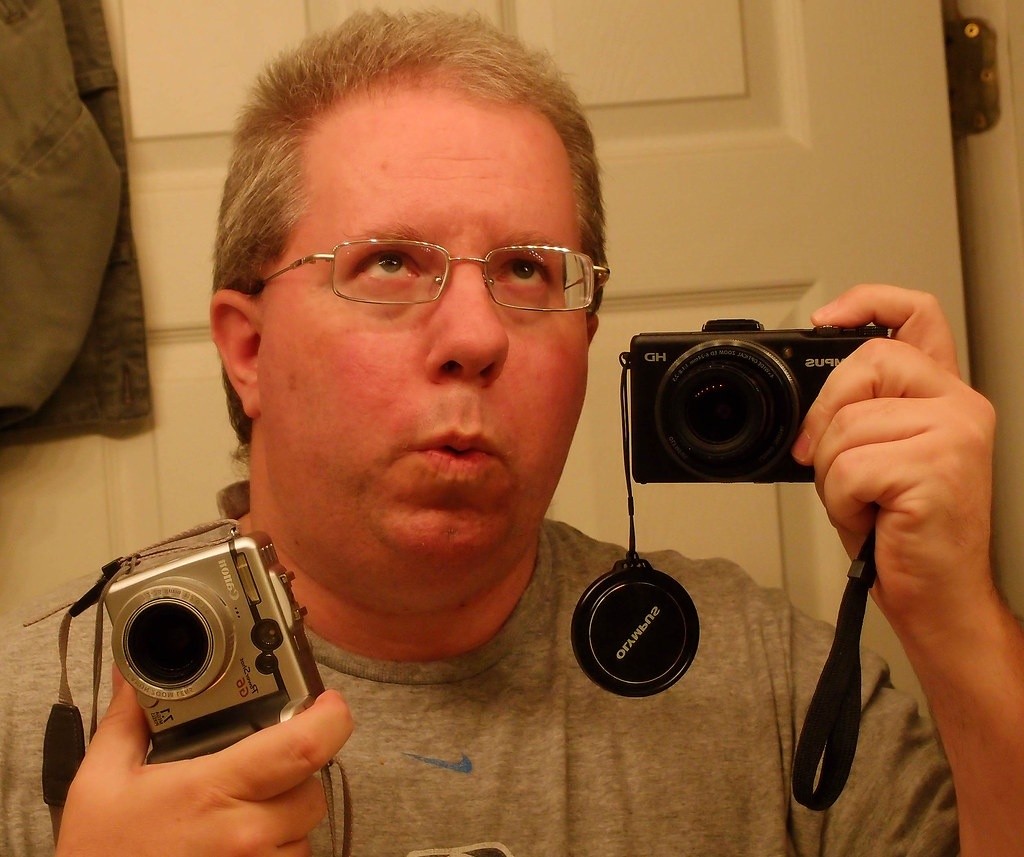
[255,239,611,313]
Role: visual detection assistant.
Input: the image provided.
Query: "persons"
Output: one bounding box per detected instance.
[0,9,1024,856]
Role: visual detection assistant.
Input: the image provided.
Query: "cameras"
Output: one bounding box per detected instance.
[97,529,328,763]
[626,317,889,483]
